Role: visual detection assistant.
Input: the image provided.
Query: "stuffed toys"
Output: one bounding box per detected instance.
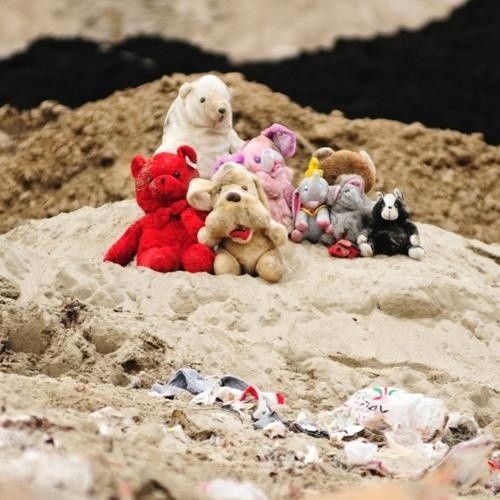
[286,170,341,243]
[186,161,289,283]
[317,171,376,244]
[151,73,245,180]
[327,240,363,258]
[356,186,425,259]
[312,145,378,192]
[211,121,298,225]
[103,144,217,275]
